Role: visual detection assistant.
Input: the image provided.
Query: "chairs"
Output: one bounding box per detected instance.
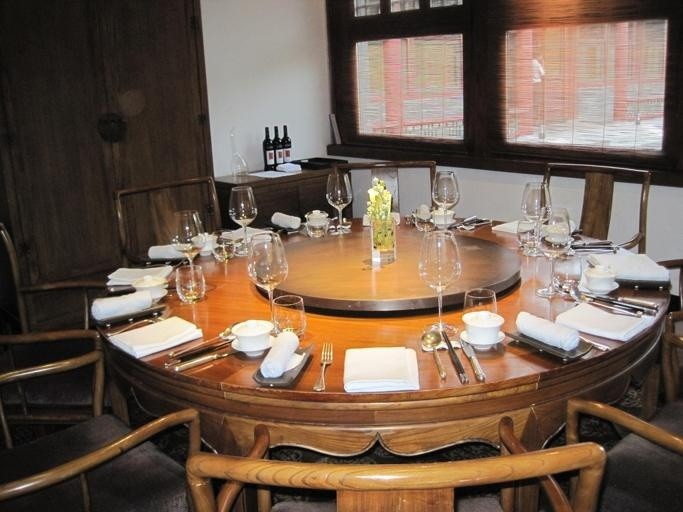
[185,417,608,511]
[658,259,683,397]
[332,161,436,218]
[539,162,652,253]
[566,333,683,512]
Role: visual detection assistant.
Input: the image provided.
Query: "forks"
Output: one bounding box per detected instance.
[314,342,336,388]
[106,306,173,337]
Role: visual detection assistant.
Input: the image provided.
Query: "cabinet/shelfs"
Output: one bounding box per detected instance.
[1,0,216,338]
[216,157,354,229]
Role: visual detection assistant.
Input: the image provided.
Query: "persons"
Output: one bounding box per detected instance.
[532,51,545,127]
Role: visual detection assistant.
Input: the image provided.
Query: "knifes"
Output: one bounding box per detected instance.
[169,329,235,371]
[457,340,488,385]
[572,290,661,319]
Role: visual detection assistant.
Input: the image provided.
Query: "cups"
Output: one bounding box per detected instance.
[462,311,506,338]
[228,319,275,345]
[463,287,499,313]
[268,294,307,336]
[303,207,329,239]
[175,265,208,304]
[212,228,236,260]
[430,209,456,222]
[582,265,614,286]
[130,278,165,292]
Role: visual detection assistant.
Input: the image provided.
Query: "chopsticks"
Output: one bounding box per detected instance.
[440,330,470,385]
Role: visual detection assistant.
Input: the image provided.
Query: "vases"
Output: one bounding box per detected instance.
[370,218,396,265]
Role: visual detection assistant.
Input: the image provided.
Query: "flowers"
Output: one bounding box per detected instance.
[366,177,396,251]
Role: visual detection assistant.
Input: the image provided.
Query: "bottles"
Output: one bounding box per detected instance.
[262,125,292,170]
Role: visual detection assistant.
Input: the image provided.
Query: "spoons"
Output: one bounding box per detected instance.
[420,330,448,382]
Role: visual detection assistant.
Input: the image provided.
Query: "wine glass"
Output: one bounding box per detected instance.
[227,185,258,257]
[418,229,461,338]
[326,174,352,234]
[170,209,206,267]
[431,170,461,208]
[249,232,289,324]
[516,179,573,302]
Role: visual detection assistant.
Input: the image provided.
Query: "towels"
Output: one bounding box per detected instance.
[92,211,307,386]
[362,212,401,227]
[343,346,421,394]
[490,219,672,352]
[245,170,303,179]
[275,162,301,173]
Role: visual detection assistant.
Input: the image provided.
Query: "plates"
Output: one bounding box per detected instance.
[230,339,274,355]
[578,280,619,295]
[460,329,507,352]
[146,290,169,303]
[429,220,457,228]
